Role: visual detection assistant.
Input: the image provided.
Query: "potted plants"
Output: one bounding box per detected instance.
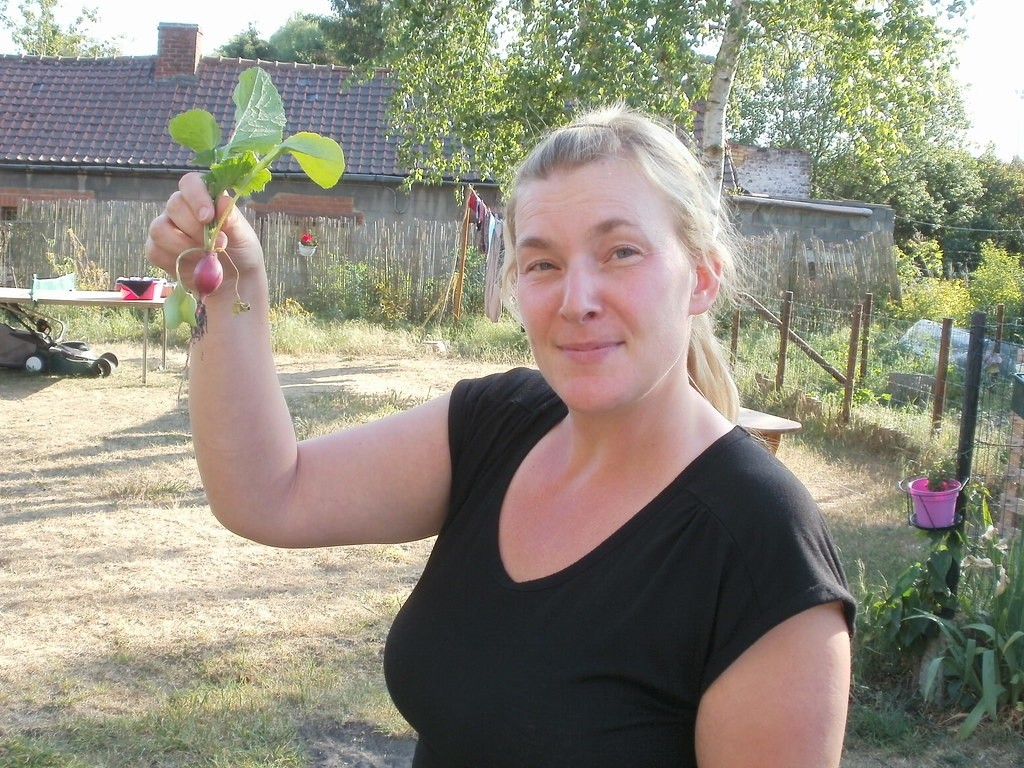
[909,458,962,528]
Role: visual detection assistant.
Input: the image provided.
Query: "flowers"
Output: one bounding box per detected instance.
[301,229,320,247]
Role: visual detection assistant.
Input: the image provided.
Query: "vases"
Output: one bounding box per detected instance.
[298,242,318,257]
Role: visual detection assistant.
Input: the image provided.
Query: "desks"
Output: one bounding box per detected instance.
[738,406,803,457]
[0,287,167,385]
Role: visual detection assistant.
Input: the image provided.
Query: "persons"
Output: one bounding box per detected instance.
[144,107,856,768]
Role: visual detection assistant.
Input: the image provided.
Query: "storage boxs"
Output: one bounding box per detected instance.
[115,276,167,300]
